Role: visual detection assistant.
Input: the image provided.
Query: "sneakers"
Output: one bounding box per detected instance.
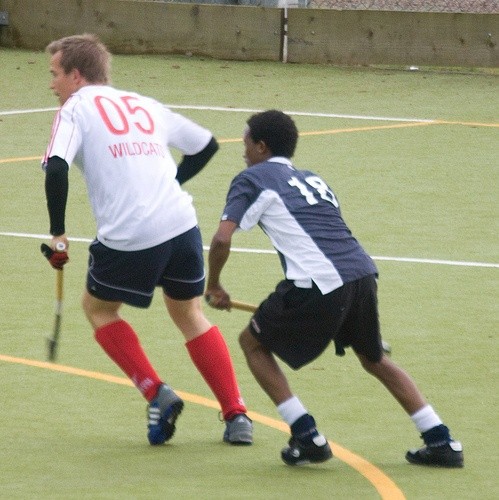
[223,416,253,444]
[405,440,464,467]
[280,436,332,466]
[146,383,184,444]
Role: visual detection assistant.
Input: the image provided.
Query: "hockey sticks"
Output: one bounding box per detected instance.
[206,293,392,353]
[48,242,65,359]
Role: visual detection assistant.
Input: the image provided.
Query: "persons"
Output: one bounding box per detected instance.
[41,34,253,445]
[206,109,464,469]
